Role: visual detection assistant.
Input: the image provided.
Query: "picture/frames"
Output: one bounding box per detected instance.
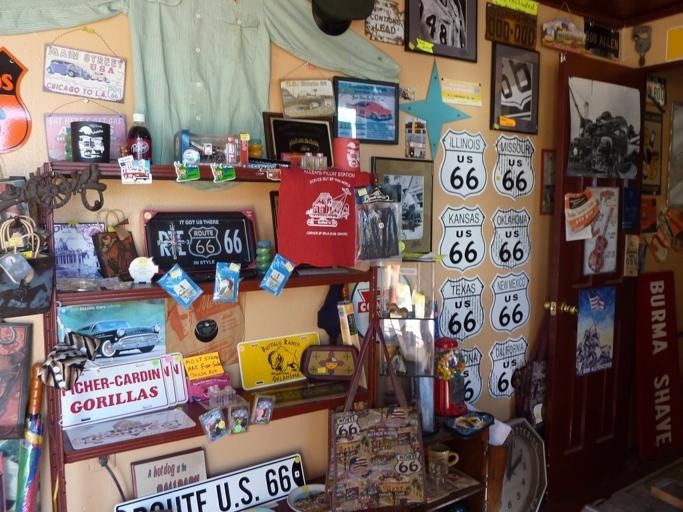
[370,156,434,255]
[403,1,478,63]
[262,110,336,170]
[489,41,540,135]
[331,75,400,145]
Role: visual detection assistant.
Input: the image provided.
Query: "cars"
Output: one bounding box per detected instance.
[344,101,392,123]
[76,317,161,358]
[295,92,326,111]
[48,60,114,85]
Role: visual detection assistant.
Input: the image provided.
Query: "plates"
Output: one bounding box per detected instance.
[285,480,328,511]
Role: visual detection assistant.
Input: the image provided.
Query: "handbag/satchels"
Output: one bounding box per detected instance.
[323,313,430,511]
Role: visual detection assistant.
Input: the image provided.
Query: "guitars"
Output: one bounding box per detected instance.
[589,207,614,274]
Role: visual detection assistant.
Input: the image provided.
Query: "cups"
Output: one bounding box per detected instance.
[426,446,461,478]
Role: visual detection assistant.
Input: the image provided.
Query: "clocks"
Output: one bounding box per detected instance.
[498,418,550,511]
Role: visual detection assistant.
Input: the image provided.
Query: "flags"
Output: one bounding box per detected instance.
[589,291,607,312]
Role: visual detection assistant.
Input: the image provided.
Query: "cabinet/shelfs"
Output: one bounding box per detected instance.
[39,161,489,512]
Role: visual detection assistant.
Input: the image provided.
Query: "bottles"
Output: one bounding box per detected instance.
[254,240,275,275]
[248,139,264,158]
[301,152,328,171]
[206,385,237,409]
[224,136,236,165]
[124,112,153,163]
